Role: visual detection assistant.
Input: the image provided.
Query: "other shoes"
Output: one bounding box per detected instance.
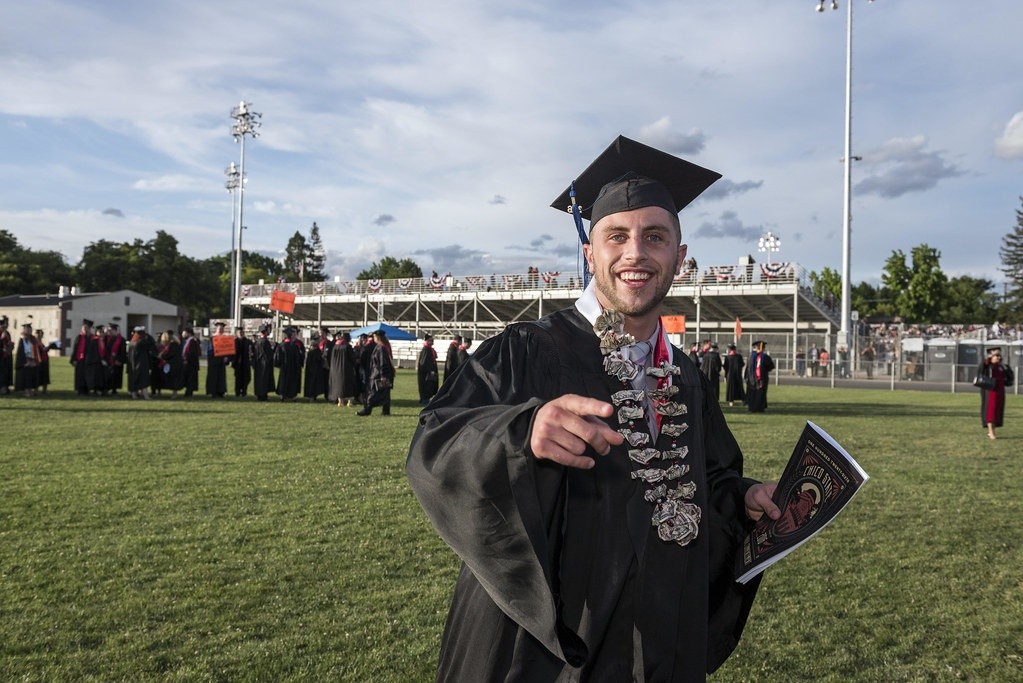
[729,402,733,406]
[381,407,390,416]
[357,407,372,416]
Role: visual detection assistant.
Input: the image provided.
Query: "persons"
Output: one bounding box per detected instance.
[688,339,776,414]
[403,134,781,682]
[69,318,396,416]
[796,321,975,381]
[417,333,439,404]
[0,315,50,396]
[977,346,1005,440]
[443,335,472,383]
[528,266,539,289]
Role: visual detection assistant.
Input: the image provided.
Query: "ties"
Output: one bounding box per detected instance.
[629,342,650,431]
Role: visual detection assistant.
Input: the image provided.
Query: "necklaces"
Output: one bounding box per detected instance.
[593,307,702,547]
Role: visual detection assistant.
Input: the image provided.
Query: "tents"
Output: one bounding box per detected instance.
[349,323,419,370]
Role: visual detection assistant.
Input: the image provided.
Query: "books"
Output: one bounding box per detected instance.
[732,419,870,585]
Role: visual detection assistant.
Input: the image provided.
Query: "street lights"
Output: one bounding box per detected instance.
[816,0,875,372]
[229,100,263,328]
[758,232,781,286]
[225,161,249,319]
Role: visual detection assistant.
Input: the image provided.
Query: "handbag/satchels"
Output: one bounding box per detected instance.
[973,375,995,390]
[375,378,394,391]
[1004,365,1013,386]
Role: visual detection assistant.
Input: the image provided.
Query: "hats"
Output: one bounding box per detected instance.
[692,342,701,346]
[549,135,723,291]
[728,344,736,350]
[0,316,472,342]
[987,348,1001,359]
[711,345,719,348]
[752,341,767,352]
[703,339,712,343]
